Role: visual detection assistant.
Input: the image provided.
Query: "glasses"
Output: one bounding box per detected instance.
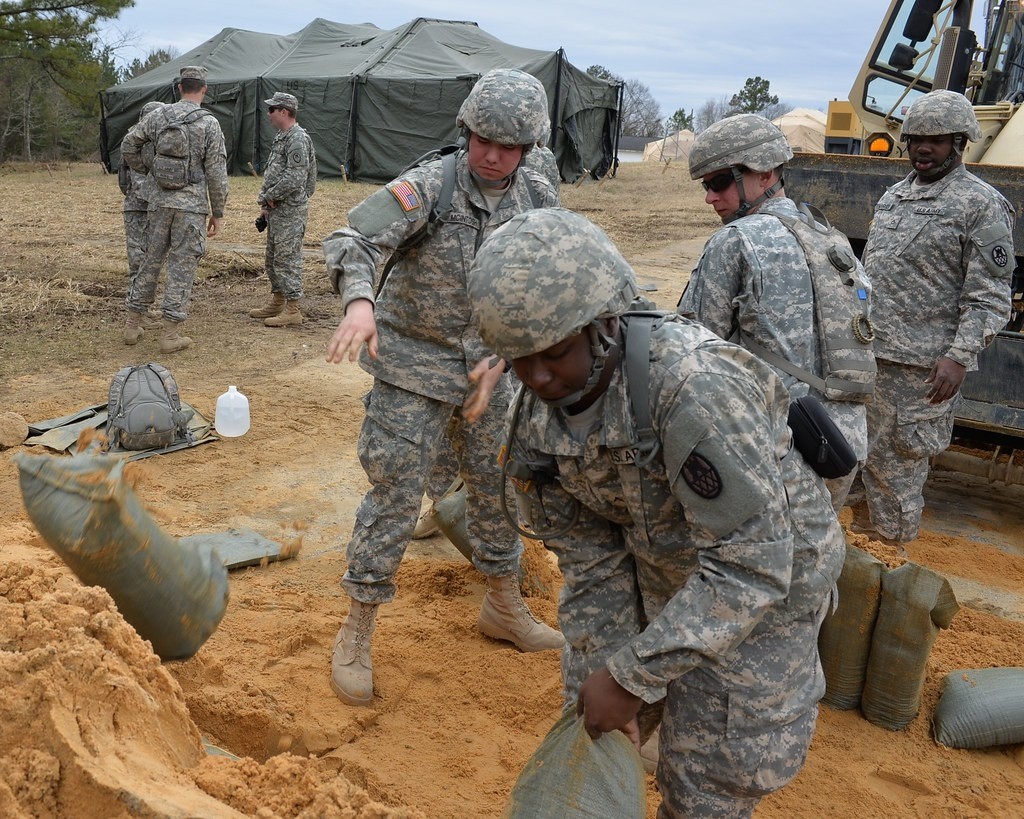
[701,166,749,192]
[268,106,283,114]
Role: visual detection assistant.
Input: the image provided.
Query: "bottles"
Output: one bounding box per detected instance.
[217,386,250,438]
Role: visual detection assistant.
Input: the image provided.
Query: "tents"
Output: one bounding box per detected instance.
[99,19,625,188]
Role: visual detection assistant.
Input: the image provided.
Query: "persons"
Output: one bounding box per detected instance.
[469,209,845,819]
[118,101,168,328]
[249,92,317,327]
[852,89,1018,548]
[123,66,229,353]
[331,70,563,705]
[672,113,865,519]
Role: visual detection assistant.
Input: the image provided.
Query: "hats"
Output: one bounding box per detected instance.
[264,92,298,110]
[180,66,208,81]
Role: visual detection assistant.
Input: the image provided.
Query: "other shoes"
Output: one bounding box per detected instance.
[410,501,439,539]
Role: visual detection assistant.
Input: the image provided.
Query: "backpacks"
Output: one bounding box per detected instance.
[103,363,187,450]
[733,201,878,405]
[151,104,213,190]
[118,157,132,195]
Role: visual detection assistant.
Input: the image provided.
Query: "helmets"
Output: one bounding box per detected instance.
[456,68,551,148]
[468,207,637,362]
[139,101,165,121]
[688,114,794,180]
[899,90,980,144]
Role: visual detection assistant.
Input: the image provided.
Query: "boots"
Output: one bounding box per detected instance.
[124,310,144,344]
[330,598,379,706]
[250,292,286,318]
[264,299,302,326]
[477,572,566,652]
[160,319,192,354]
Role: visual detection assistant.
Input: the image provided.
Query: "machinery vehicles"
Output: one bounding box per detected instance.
[781,0,1024,488]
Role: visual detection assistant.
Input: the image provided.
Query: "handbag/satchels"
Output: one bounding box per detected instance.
[786,393,856,479]
